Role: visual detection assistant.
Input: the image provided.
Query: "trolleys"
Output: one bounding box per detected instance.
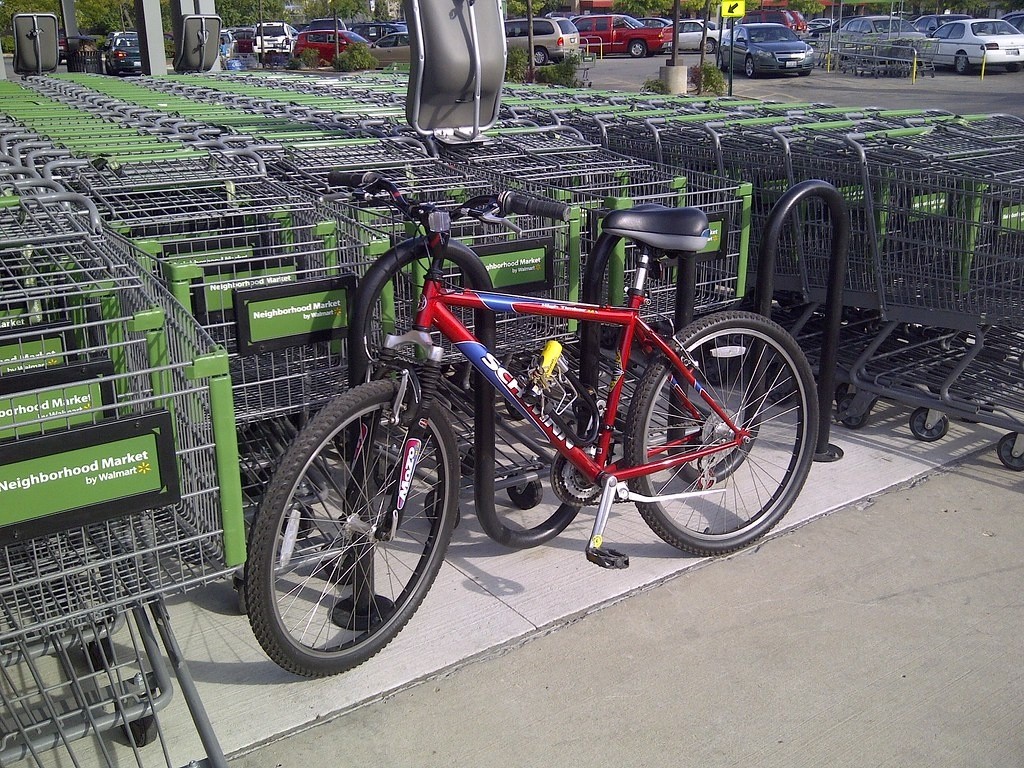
[0,35,1024,766]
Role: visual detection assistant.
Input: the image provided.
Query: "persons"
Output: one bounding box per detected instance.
[219,38,228,70]
[769,28,779,40]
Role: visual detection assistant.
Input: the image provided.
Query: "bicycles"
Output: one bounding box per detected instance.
[238,169,822,682]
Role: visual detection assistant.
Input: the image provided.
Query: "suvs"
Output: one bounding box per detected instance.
[784,9,808,34]
[350,22,408,43]
[308,18,348,31]
[252,20,299,62]
[726,8,797,36]
[506,18,581,67]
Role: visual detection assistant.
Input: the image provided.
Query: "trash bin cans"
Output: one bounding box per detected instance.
[64,36,103,74]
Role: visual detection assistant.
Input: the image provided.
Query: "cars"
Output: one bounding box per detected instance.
[985,10,1024,35]
[221,27,254,54]
[838,16,926,49]
[569,15,674,57]
[913,15,974,39]
[375,30,412,50]
[105,33,144,74]
[661,20,732,54]
[809,14,884,38]
[293,30,381,70]
[809,18,834,30]
[922,19,1023,74]
[717,23,815,78]
[637,16,673,30]
